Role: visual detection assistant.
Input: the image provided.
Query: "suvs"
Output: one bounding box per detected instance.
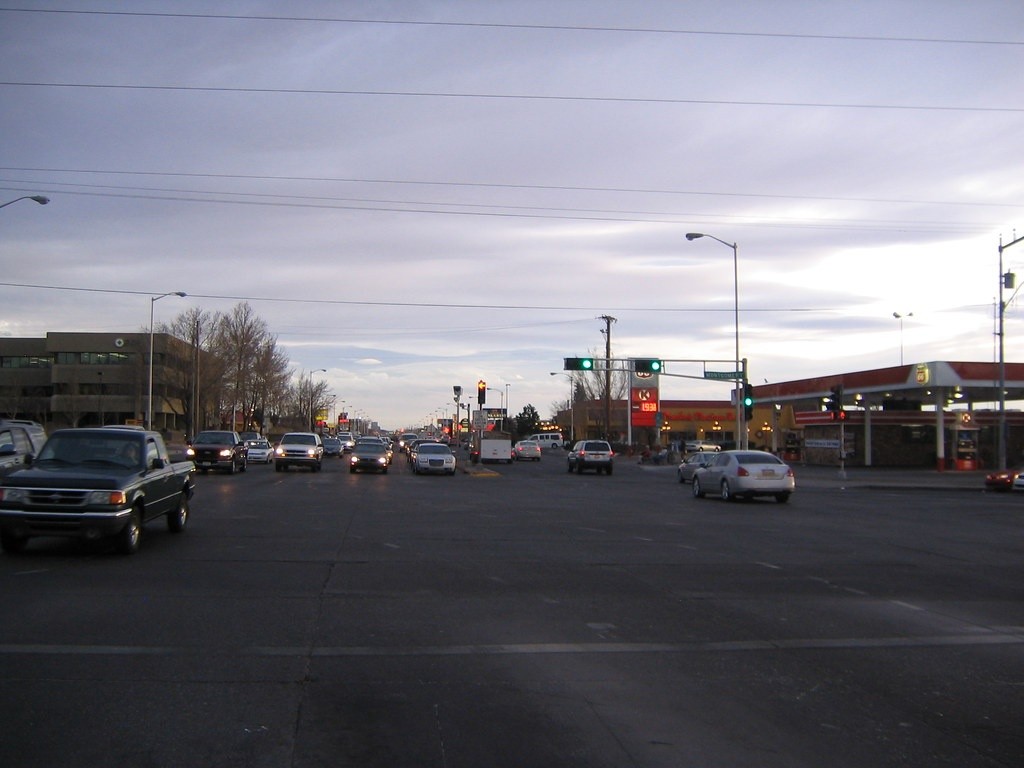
[0,419,48,477]
[567,440,613,476]
[337,435,356,451]
[240,432,261,442]
[273,432,323,473]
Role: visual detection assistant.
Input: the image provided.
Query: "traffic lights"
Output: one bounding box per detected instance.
[477,382,486,405]
[831,384,839,412]
[566,358,593,370]
[744,384,754,420]
[635,360,662,373]
[836,411,845,421]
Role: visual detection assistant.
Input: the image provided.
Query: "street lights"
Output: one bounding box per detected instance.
[686,232,740,451]
[434,410,444,428]
[762,422,770,452]
[893,312,914,367]
[308,369,326,432]
[342,406,353,432]
[418,412,438,440]
[487,388,503,432]
[147,292,188,430]
[662,421,671,446]
[438,407,448,428]
[550,372,573,442]
[333,401,346,438]
[354,408,363,434]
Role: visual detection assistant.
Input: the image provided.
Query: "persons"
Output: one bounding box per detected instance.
[680,440,686,462]
[114,443,139,466]
[639,445,650,464]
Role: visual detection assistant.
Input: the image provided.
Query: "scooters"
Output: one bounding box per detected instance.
[471,451,479,463]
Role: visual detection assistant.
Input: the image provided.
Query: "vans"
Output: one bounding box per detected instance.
[528,433,564,449]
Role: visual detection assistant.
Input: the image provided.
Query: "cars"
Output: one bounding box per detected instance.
[322,438,344,459]
[185,430,248,474]
[984,462,1024,494]
[411,443,457,476]
[349,442,389,474]
[317,430,473,465]
[245,439,274,465]
[514,440,541,462]
[677,450,717,484]
[686,439,721,454]
[691,449,795,503]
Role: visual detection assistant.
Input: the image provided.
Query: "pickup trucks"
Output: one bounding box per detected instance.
[0,425,197,560]
